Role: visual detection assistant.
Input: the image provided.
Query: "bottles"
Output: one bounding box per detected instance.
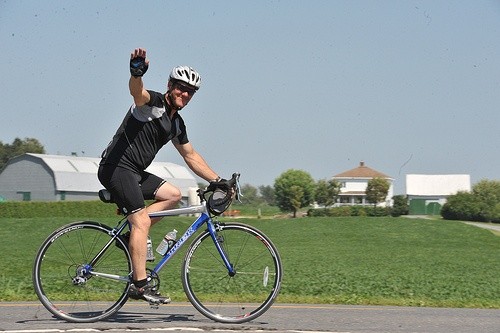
[156,229,178,255]
[146,235,153,258]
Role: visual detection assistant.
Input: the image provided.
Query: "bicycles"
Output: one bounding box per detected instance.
[31,171,283,325]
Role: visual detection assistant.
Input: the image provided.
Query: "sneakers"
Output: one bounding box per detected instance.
[116,230,156,261]
[127,281,171,304]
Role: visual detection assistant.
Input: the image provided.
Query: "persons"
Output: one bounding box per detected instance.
[98,48,234,303]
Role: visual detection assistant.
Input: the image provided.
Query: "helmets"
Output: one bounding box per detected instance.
[169,65,201,90]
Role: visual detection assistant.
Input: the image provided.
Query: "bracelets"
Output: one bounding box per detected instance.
[215,176,220,182]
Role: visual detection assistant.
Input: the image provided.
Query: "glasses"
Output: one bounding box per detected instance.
[172,81,196,95]
